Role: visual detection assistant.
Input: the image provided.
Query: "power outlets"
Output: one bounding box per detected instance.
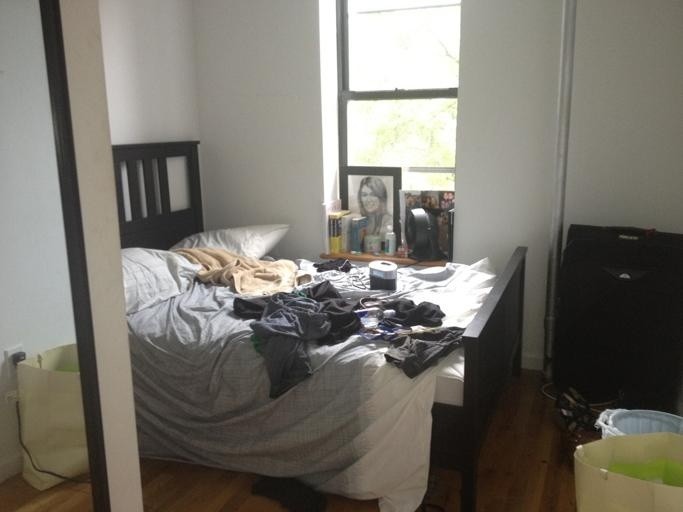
[4,345,24,379]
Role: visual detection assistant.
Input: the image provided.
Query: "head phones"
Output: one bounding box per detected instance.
[355,296,397,319]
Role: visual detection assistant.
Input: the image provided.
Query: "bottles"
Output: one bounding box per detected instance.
[355,307,396,327]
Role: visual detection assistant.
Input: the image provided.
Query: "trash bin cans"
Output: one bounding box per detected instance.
[594,408,683,439]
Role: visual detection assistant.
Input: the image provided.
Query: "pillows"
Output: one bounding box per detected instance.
[121,247,202,313]
[169,217,289,260]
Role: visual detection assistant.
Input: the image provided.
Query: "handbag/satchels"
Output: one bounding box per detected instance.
[14,343,89,492]
[571,432,683,511]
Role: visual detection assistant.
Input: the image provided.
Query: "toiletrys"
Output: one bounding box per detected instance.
[363,235,382,252]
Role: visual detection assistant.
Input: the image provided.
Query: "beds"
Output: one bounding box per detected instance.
[110,141,528,512]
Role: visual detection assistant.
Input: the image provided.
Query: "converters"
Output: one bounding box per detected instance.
[13,351,26,366]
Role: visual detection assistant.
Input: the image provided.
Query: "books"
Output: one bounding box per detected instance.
[327,208,367,253]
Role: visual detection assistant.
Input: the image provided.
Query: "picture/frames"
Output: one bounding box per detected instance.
[339,166,402,251]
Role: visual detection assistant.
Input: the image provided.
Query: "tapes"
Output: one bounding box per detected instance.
[365,235,382,251]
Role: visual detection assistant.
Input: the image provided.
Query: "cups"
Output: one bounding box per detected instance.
[364,235,382,255]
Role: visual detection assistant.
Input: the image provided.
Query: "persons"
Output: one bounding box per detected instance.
[355,176,393,234]
[404,192,454,225]
